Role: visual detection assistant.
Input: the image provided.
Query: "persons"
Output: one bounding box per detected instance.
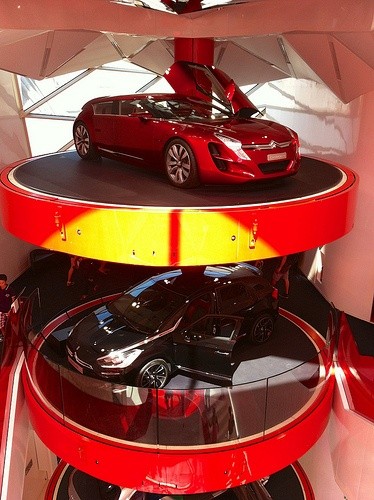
[0,273,15,359]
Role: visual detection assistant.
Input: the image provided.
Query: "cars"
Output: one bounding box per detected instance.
[63,259,280,391]
[96,475,273,500]
[71,60,301,194]
